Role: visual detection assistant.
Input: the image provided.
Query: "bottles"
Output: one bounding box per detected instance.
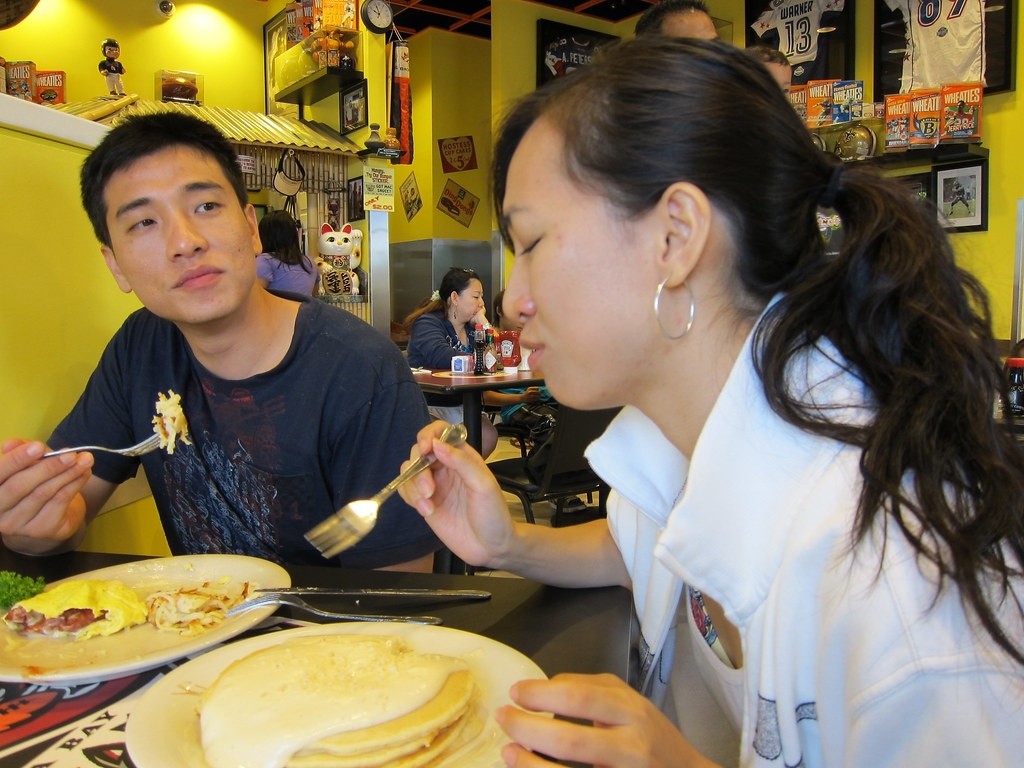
[499,330,521,374]
[485,329,498,372]
[472,324,487,376]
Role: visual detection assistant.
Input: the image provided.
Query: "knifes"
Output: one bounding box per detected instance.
[254,587,492,601]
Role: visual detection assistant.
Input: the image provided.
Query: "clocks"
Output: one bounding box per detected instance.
[361,1,393,33]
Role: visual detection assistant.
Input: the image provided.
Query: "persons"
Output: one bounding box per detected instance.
[633,0,719,39]
[740,46,792,101]
[255,210,322,296]
[0,113,448,578]
[98,39,127,96]
[348,183,364,220]
[949,181,971,215]
[345,100,359,124]
[481,289,587,512]
[401,267,500,461]
[398,37,1024,768]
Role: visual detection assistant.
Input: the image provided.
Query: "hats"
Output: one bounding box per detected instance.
[273,149,306,197]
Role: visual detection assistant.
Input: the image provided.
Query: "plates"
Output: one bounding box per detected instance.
[0,553,292,688]
[123,621,556,768]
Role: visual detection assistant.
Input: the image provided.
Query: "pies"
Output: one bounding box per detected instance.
[198,633,483,768]
[2,579,150,641]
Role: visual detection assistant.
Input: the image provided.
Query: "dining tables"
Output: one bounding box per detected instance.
[409,367,547,559]
[1,550,638,768]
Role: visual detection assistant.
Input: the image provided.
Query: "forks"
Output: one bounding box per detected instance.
[303,423,468,559]
[43,431,160,456]
[224,592,443,626]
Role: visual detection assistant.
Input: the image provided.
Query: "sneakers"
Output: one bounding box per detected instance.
[548,495,586,513]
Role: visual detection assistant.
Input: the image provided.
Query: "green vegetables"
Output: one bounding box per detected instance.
[0,570,46,611]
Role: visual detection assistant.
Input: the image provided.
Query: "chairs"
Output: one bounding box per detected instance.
[483,407,623,526]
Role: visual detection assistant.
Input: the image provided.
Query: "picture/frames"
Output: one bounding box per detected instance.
[932,158,987,233]
[346,176,365,221]
[338,81,369,136]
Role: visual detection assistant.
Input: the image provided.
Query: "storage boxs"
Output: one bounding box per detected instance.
[883,84,983,147]
[786,78,864,127]
[5,61,36,103]
[36,70,66,106]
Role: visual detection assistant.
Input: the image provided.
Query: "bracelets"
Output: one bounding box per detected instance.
[482,322,492,330]
